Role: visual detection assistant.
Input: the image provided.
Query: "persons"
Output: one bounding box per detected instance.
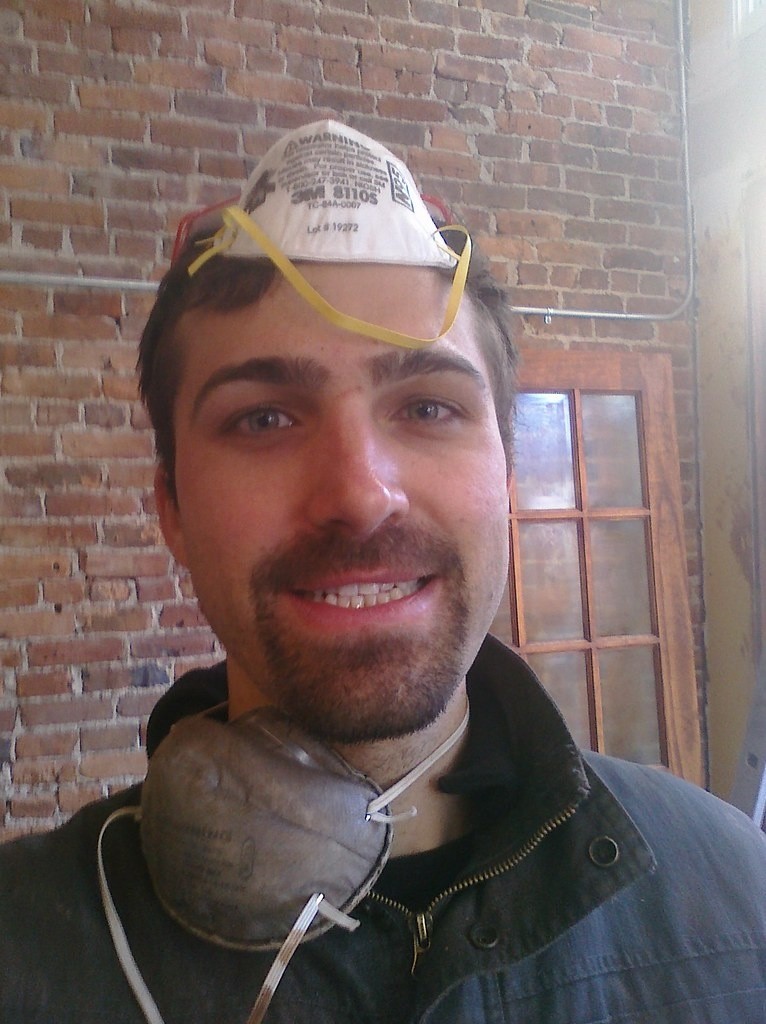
[0,120,766,1024]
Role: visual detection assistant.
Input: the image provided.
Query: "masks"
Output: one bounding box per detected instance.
[90,698,474,1024]
[185,115,479,354]
